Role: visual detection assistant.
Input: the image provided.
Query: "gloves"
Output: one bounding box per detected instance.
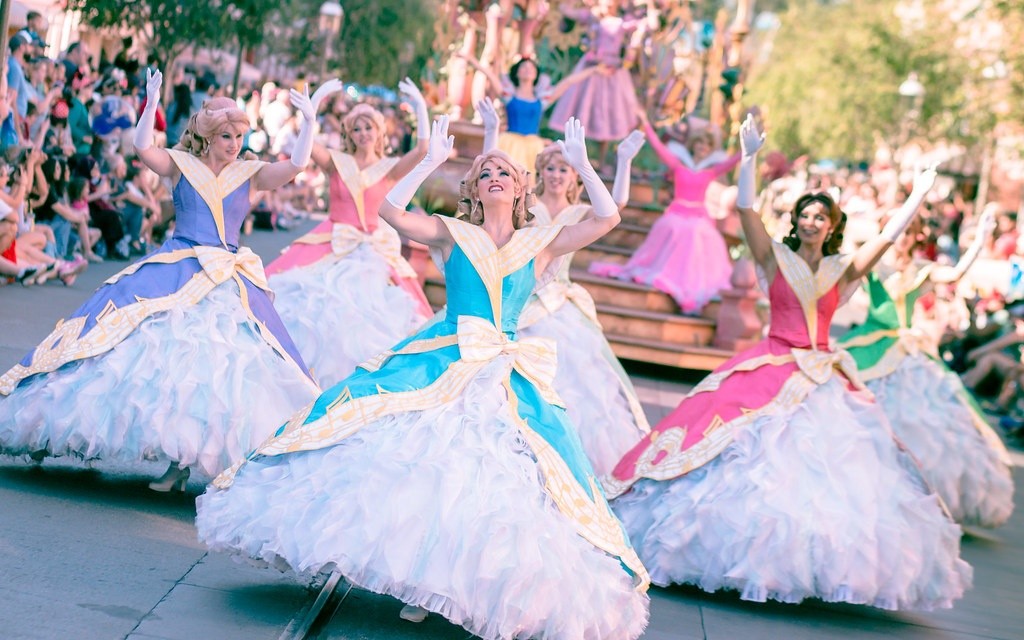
[290,82,315,167]
[557,116,619,217]
[398,77,430,139]
[881,162,937,244]
[476,96,500,154]
[737,112,766,208]
[957,209,997,272]
[612,130,645,203]
[133,67,163,149]
[386,114,455,210]
[301,78,342,140]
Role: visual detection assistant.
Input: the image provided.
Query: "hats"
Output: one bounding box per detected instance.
[73,67,95,89]
[52,93,74,117]
[8,30,39,47]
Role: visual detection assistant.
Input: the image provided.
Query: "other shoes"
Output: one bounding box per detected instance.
[128,241,146,256]
[59,260,87,278]
[21,264,46,286]
[85,252,102,263]
[399,604,428,622]
[107,248,129,261]
[36,260,60,285]
[64,254,82,285]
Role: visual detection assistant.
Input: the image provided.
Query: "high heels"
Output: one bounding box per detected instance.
[149,466,190,491]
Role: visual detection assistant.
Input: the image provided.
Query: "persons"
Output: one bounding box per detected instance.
[116,68,318,492]
[476,97,646,484]
[299,76,431,392]
[379,112,622,640]
[828,187,996,541]
[0,0,767,288]
[757,142,1024,452]
[734,112,938,614]
